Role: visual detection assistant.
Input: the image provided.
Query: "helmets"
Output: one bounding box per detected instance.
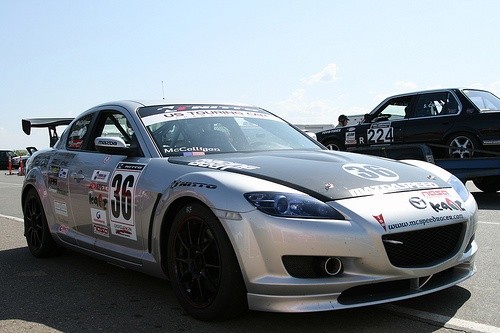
[337,114,350,126]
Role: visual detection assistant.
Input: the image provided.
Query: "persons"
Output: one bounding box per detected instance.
[336,114,351,127]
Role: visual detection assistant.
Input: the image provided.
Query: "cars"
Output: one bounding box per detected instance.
[316,88,500,159]
[20,100,478,320]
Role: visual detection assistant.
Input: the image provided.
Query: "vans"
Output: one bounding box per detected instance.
[0,147,38,175]
